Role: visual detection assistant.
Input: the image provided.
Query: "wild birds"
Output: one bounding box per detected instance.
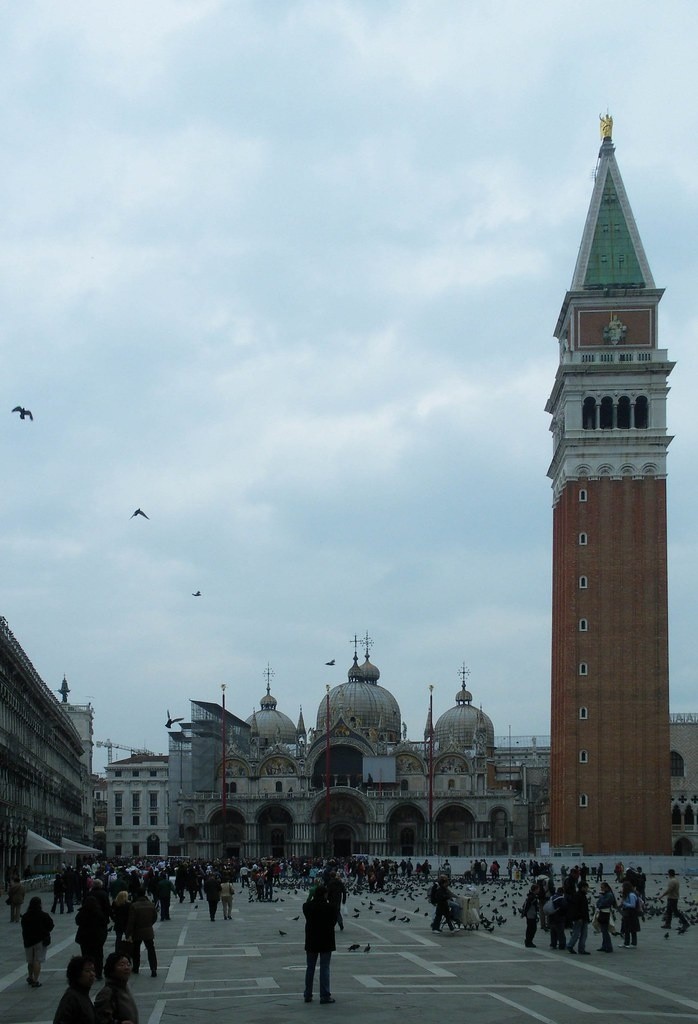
[192,591,201,596]
[325,659,335,665]
[130,508,150,520]
[165,709,184,729]
[12,406,33,421]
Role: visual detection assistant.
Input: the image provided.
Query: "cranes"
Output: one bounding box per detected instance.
[95,739,156,765]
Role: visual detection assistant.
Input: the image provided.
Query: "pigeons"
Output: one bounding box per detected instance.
[195,873,698,954]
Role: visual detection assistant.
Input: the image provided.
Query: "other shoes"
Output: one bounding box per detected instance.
[661,925,671,930]
[152,970,157,977]
[682,923,690,930]
[526,942,537,948]
[451,928,460,934]
[550,943,558,949]
[567,946,578,954]
[432,929,440,934]
[340,925,344,930]
[320,997,335,1004]
[305,998,312,1003]
[597,947,611,952]
[228,916,232,919]
[27,977,32,984]
[32,982,42,988]
[67,911,71,913]
[132,967,139,974]
[224,916,227,920]
[618,944,629,947]
[626,945,637,949]
[51,910,55,914]
[579,951,591,954]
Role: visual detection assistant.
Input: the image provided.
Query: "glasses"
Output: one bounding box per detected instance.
[83,967,95,973]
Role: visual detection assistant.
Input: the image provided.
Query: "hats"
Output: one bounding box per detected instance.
[441,874,451,881]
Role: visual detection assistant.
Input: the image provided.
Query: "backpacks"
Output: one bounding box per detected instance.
[628,892,645,913]
[543,896,565,915]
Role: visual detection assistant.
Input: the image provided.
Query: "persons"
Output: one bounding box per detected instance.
[7,854,691,1024]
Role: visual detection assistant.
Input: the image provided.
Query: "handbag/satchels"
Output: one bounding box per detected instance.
[6,898,11,905]
